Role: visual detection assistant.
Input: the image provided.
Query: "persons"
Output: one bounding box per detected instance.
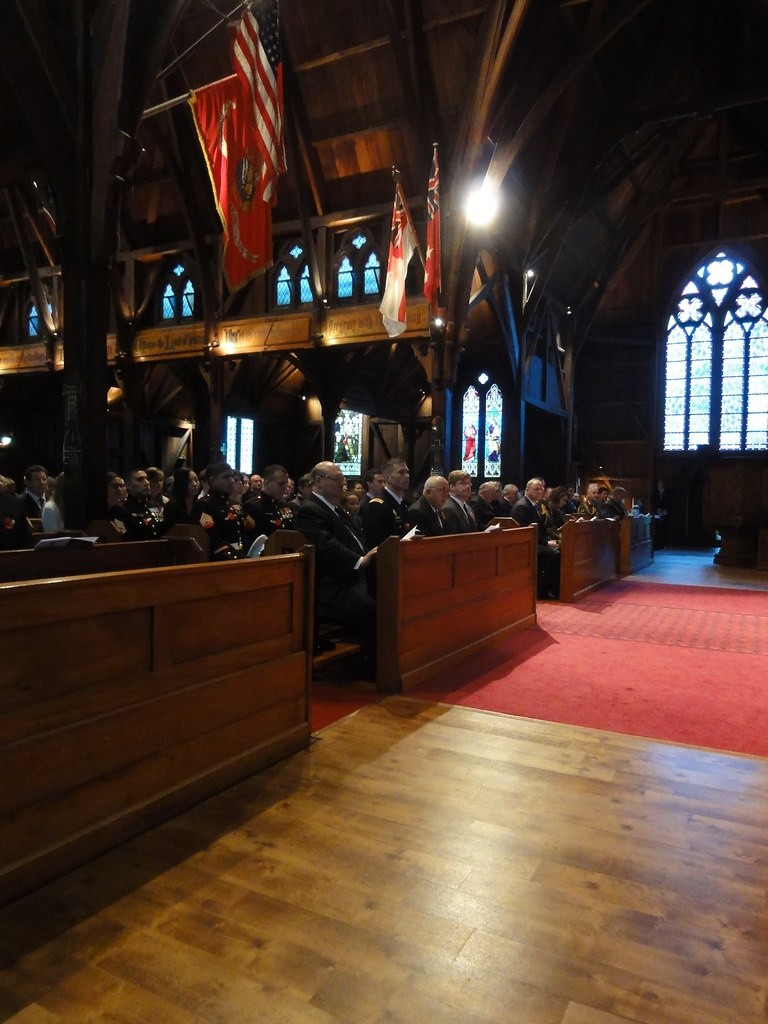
[0,458,630,676]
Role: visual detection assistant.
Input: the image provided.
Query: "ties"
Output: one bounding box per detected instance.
[463,504,479,532]
[335,507,368,554]
[535,505,542,520]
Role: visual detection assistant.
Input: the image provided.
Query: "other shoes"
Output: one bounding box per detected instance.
[313,636,335,656]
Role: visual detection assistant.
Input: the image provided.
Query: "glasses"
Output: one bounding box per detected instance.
[320,475,347,485]
[459,481,473,486]
[429,487,453,495]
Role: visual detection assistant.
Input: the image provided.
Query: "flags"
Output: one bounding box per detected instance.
[189,78,274,293]
[378,191,417,338]
[226,0,285,179]
[424,150,441,316]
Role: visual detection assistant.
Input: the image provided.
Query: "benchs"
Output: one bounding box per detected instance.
[264,517,520,667]
[0,516,203,584]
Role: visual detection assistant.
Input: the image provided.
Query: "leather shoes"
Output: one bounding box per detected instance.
[540,590,560,600]
